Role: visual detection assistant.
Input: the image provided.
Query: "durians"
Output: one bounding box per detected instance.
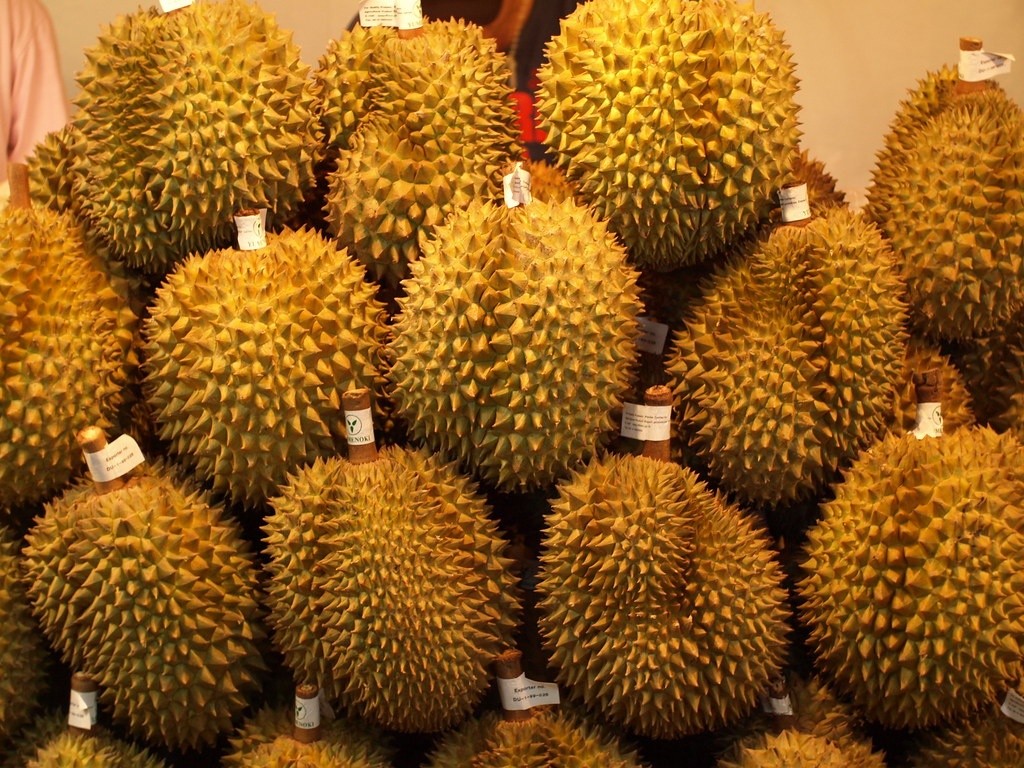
[0,0,1024,768]
